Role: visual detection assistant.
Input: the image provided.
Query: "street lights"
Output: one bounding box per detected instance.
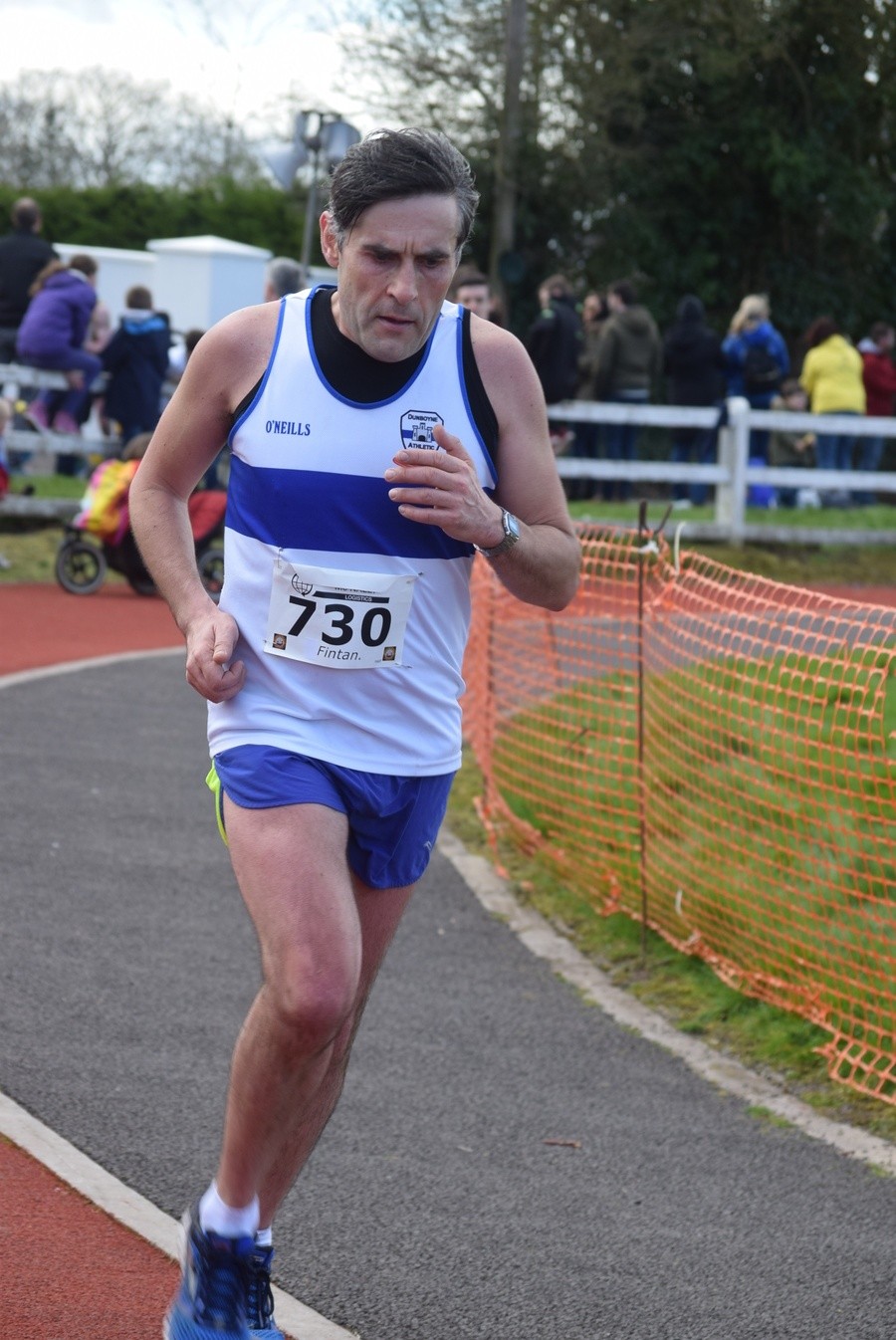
[265,107,361,277]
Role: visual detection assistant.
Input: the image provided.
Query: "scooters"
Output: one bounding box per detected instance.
[56,457,226,605]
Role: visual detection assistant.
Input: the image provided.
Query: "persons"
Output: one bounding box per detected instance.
[127,132,584,1340]
[0,197,311,491]
[450,264,896,509]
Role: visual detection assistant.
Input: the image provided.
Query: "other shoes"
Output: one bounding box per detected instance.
[23,403,52,436]
[54,413,78,434]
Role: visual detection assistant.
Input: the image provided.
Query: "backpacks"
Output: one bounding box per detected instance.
[739,330,785,393]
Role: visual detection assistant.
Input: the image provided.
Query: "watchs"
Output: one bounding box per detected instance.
[473,504,521,560]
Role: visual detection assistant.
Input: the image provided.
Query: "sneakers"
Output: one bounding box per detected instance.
[160,1188,288,1340]
